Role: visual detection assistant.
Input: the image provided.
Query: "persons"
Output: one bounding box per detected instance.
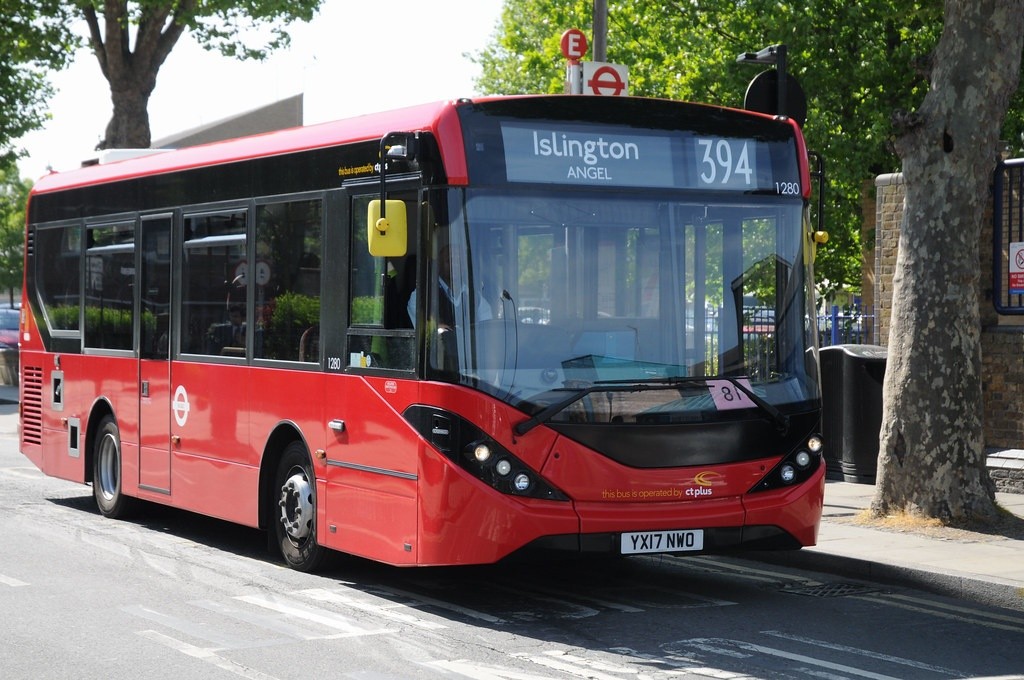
[407,244,500,389]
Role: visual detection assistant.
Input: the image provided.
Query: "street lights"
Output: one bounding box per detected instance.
[737,44,789,114]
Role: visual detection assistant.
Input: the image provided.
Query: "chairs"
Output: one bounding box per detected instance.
[298,326,319,361]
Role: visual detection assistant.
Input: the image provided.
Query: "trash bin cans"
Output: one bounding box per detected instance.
[819,343,888,483]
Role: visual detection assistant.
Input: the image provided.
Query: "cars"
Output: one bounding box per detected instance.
[0,301,21,403]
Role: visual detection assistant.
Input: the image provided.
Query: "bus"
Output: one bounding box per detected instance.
[14,92,830,576]
